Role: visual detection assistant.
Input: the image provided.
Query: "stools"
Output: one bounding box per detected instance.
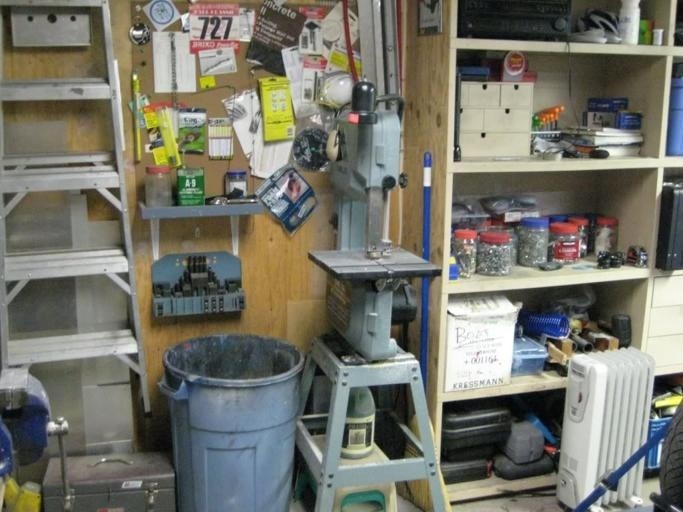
[290,337,445,512]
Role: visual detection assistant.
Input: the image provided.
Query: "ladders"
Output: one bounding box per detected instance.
[0,0,153,418]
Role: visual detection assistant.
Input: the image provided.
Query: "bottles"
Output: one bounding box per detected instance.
[144,164,173,210]
[451,213,619,277]
[223,170,248,198]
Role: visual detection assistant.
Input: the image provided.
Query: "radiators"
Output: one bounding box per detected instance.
[556,347,655,511]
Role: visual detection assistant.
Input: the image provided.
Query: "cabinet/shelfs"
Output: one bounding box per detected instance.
[637,0,682,377]
[395,1,674,502]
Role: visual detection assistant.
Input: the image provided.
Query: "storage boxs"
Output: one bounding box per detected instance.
[646,417,672,472]
[443,294,517,392]
[668,74,683,159]
[655,180,683,273]
[441,409,553,485]
[510,335,547,377]
[42,451,176,512]
[457,70,536,159]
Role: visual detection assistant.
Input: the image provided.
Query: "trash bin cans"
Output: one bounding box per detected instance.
[158,336,305,512]
